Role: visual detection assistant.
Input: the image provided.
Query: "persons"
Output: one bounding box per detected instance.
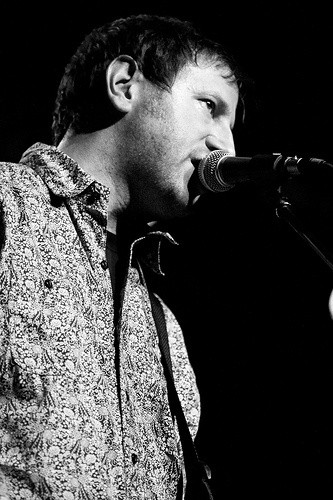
[1,14,247,500]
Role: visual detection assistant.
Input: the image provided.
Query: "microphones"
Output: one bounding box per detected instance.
[198,149,329,194]
